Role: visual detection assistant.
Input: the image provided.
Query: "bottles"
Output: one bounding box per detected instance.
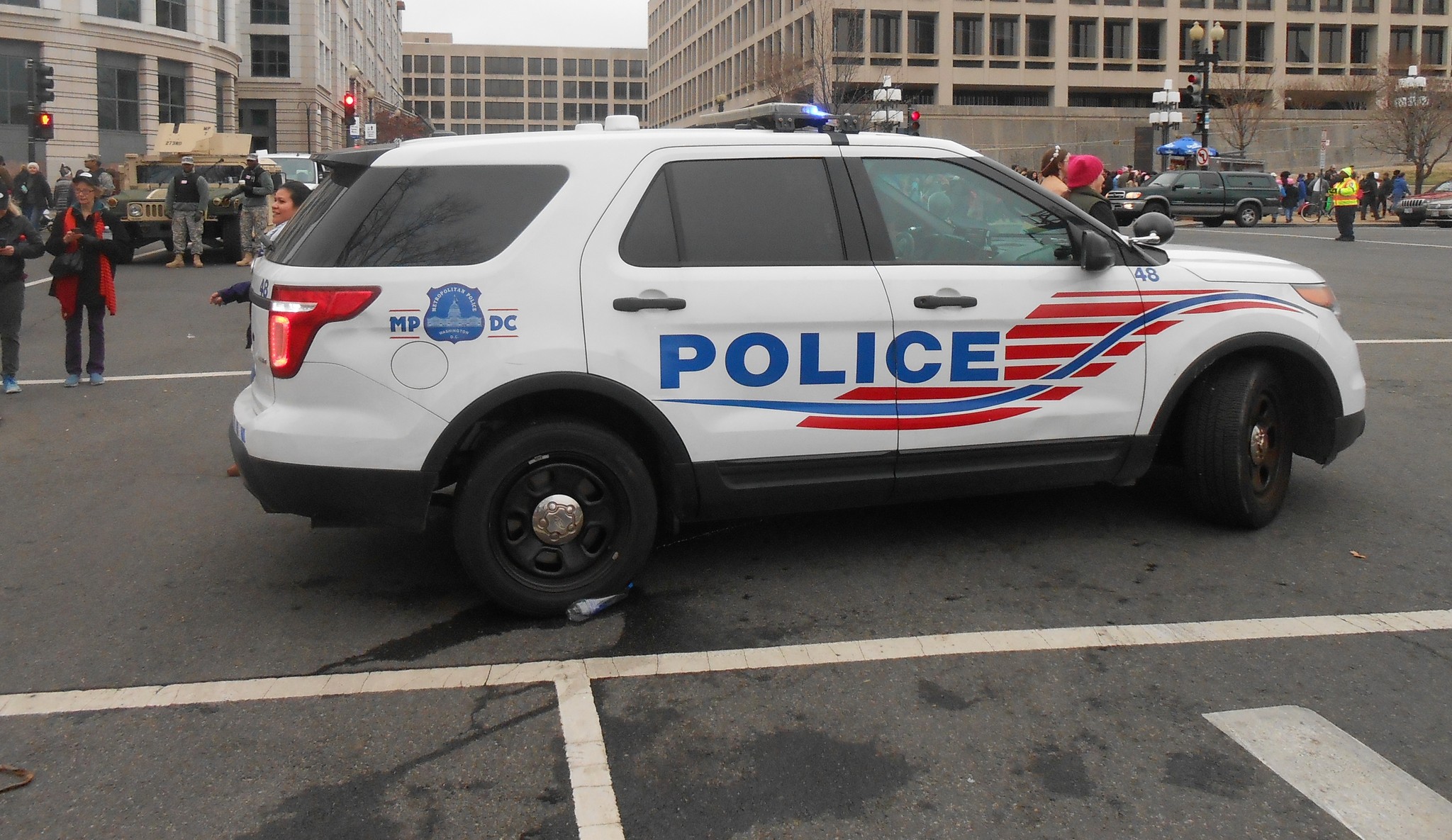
[19,234,29,245]
[102,225,113,241]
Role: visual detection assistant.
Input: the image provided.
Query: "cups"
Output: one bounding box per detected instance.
[239,180,246,186]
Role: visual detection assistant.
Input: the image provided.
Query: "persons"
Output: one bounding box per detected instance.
[222,153,275,266]
[163,156,210,269]
[1060,155,1121,234]
[0,181,46,394]
[0,154,115,234]
[883,165,1159,224]
[44,172,130,387]
[1327,167,1359,242]
[1038,145,1072,196]
[208,180,313,478]
[1270,164,1412,224]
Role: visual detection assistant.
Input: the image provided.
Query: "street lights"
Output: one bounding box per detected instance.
[344,63,359,147]
[1149,79,1182,172]
[392,107,401,140]
[869,76,903,133]
[1189,21,1224,170]
[365,86,378,145]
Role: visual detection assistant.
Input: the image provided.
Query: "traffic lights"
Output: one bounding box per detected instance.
[344,90,356,125]
[33,61,55,105]
[1191,112,1203,123]
[1186,71,1202,106]
[907,107,921,136]
[37,113,55,139]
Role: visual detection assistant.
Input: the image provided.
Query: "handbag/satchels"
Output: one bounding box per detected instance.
[49,249,84,278]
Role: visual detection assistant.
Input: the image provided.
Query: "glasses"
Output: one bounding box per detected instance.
[1102,174,1105,175]
[72,187,96,194]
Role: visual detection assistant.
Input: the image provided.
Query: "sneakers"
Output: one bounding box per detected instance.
[63,373,104,386]
[3,376,22,392]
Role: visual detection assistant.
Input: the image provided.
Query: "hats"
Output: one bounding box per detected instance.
[1341,167,1352,177]
[1066,155,1102,187]
[181,157,193,165]
[1012,164,1020,168]
[246,153,259,161]
[1,156,6,166]
[60,153,102,185]
[1271,172,1295,184]
[1117,165,1133,174]
[1141,172,1150,180]
[27,162,40,171]
[1330,165,1336,168]
[1,180,9,210]
[1299,173,1304,178]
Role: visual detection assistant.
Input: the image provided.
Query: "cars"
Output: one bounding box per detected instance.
[1426,196,1451,229]
[251,149,330,193]
[1394,180,1451,226]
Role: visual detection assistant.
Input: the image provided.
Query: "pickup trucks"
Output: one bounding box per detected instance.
[1106,168,1280,227]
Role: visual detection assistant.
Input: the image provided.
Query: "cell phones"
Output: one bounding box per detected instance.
[0,238,7,248]
[71,228,81,235]
[21,185,28,192]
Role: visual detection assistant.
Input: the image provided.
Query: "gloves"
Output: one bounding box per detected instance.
[242,185,253,192]
[223,193,231,200]
[164,211,173,219]
[193,211,202,221]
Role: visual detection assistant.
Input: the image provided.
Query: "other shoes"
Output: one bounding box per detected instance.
[1286,219,1293,222]
[1337,234,1355,242]
[1361,212,1396,220]
[1271,218,1276,222]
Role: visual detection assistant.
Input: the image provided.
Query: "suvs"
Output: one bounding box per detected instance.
[227,103,1368,621]
[103,123,285,257]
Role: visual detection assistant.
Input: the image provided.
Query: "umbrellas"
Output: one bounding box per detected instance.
[1155,136,1221,170]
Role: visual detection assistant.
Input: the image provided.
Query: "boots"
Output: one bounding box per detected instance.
[166,254,204,268]
[237,252,258,266]
